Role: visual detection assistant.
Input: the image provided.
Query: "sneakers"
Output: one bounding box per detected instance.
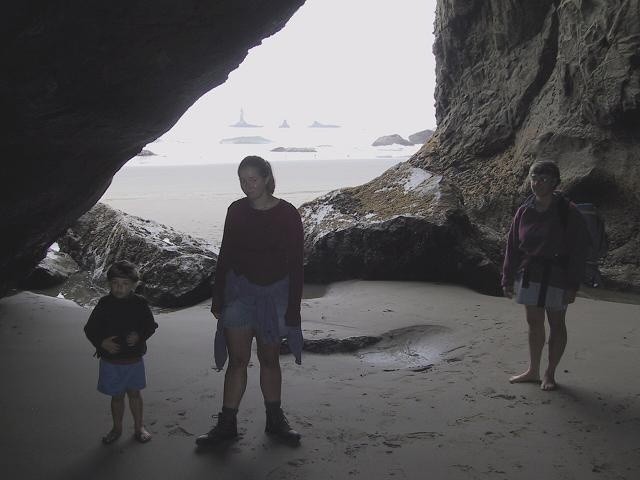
[196,413,237,447]
[265,408,301,442]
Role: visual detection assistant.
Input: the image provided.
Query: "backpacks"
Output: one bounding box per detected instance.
[526,195,609,266]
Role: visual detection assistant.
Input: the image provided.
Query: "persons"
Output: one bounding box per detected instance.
[83,261,159,445]
[193,154,305,446]
[498,159,589,392]
